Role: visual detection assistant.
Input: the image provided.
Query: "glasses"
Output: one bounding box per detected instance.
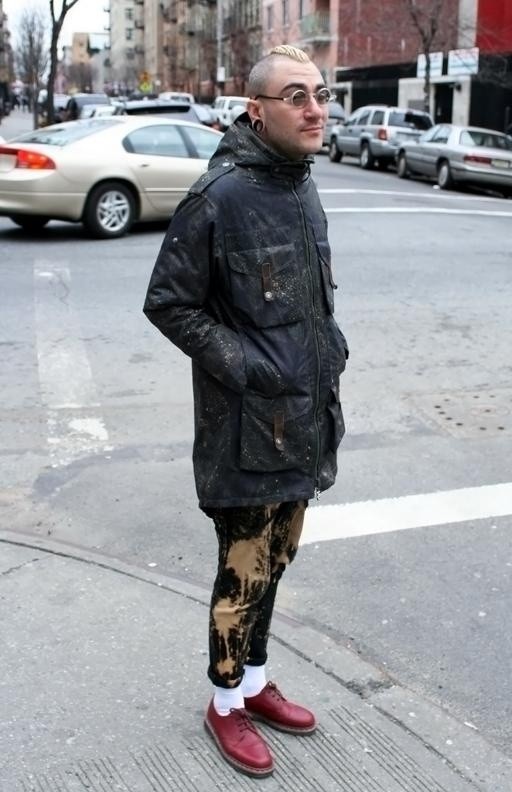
[248,88,337,108]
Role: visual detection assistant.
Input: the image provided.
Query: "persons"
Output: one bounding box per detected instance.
[143,44,354,778]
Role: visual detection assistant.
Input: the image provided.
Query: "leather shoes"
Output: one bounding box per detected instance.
[236,681,318,755]
[203,692,277,778]
